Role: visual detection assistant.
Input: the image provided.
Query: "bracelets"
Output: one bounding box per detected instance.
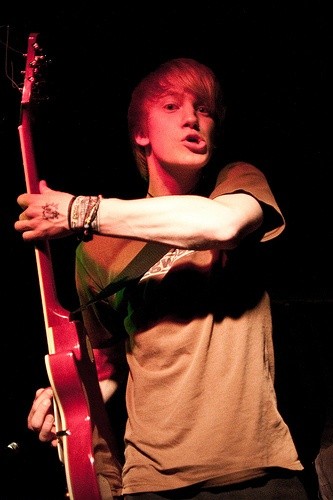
[68,193,104,238]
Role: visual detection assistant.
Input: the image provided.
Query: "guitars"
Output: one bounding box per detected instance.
[15,31,124,500]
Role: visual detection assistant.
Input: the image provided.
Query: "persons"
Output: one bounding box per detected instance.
[15,50,311,500]
